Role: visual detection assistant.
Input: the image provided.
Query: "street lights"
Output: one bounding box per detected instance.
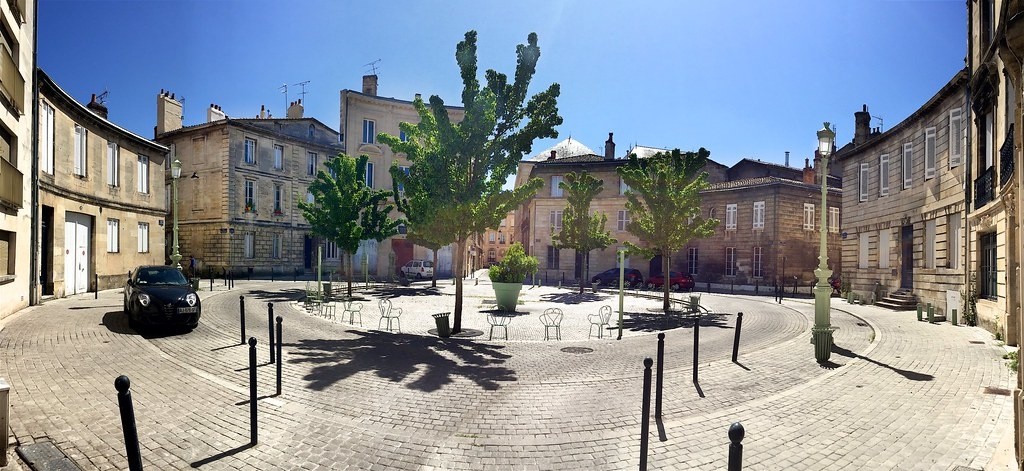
[171,158,183,271]
[810,122,835,362]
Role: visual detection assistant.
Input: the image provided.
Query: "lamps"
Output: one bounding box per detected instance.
[190,172,199,180]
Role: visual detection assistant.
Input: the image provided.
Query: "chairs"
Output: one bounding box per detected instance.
[486,305,512,340]
[306,277,340,319]
[340,276,403,294]
[596,279,701,332]
[377,299,403,332]
[539,307,564,341]
[340,293,363,327]
[587,305,612,339]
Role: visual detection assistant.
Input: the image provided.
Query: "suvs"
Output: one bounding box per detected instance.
[400,259,433,279]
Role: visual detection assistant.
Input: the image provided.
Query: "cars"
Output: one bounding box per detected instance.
[646,271,695,291]
[592,268,643,287]
[124,265,201,330]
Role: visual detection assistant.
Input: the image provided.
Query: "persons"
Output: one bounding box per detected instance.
[399,271,410,287]
[189,254,196,277]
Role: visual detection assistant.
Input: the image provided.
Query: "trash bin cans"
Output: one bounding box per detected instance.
[690,296,701,312]
[430,313,451,339]
[591,283,599,293]
[322,284,331,295]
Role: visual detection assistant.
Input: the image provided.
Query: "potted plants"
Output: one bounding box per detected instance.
[247,202,253,211]
[489,241,540,312]
[276,207,281,215]
